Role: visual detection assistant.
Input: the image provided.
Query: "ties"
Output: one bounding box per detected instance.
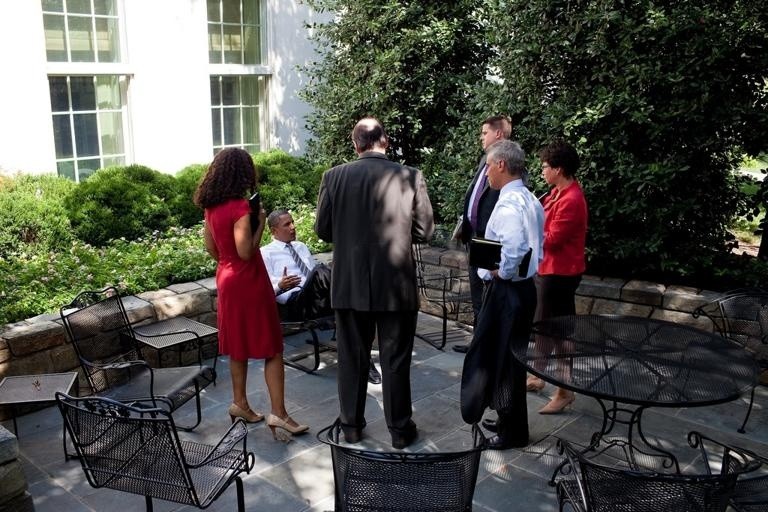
[283,241,310,281]
[470,164,488,238]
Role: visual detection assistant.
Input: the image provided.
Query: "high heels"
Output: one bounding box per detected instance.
[264,413,311,441]
[226,401,263,427]
[537,393,576,416]
[526,376,545,392]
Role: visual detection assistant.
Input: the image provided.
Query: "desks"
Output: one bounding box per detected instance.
[120,315,221,386]
[508,313,762,485]
[0,371,80,462]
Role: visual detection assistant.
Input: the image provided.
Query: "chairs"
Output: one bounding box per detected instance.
[312,414,489,512]
[678,289,768,434]
[55,390,255,512]
[278,317,335,374]
[60,286,216,432]
[556,430,761,512]
[414,238,474,351]
[730,445,768,512]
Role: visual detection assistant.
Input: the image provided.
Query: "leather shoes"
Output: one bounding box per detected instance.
[481,417,529,450]
[452,342,472,353]
[369,360,382,385]
[391,423,418,450]
[341,428,366,444]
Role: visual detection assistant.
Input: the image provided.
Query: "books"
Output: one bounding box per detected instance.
[468,237,532,278]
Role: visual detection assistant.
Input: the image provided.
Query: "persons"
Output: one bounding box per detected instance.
[526,140,588,415]
[479,139,546,451]
[312,117,436,450]
[194,147,310,443]
[452,114,511,353]
[258,210,382,385]
